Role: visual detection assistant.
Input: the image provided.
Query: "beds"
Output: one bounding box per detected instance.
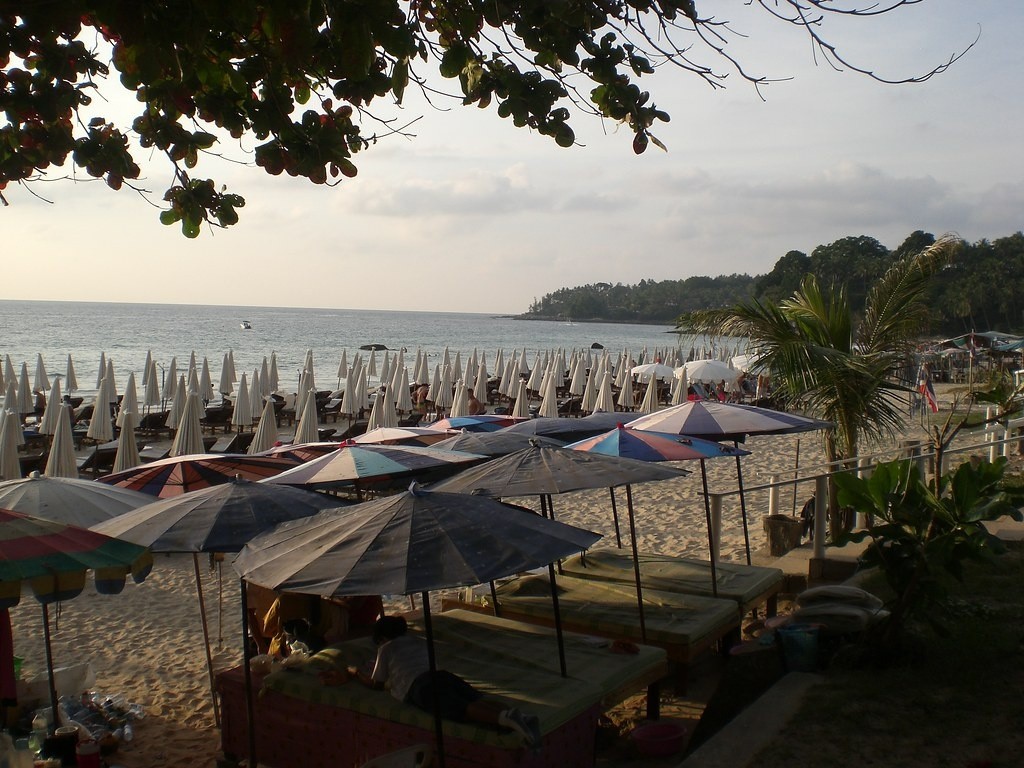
[215,546,782,767]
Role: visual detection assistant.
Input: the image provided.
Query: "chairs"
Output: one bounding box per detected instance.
[15,367,754,479]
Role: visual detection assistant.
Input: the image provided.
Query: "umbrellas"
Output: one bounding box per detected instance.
[0,326,1024,768]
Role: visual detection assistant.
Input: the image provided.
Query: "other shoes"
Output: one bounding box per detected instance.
[500,707,541,756]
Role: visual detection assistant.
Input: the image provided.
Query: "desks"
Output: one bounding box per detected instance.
[931,371,950,382]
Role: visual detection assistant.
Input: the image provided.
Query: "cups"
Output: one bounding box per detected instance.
[474,594,481,603]
[458,592,464,601]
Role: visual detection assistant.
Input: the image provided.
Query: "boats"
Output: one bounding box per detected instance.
[240,320,251,329]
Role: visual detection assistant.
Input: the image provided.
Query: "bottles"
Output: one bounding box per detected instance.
[465,585,472,602]
[0,690,146,768]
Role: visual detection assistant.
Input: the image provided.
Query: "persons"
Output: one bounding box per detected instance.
[63,395,73,423]
[21,388,46,426]
[718,380,726,390]
[411,383,429,421]
[468,389,484,415]
[202,383,215,406]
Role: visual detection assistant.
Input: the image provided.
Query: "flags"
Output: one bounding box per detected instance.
[917,358,939,415]
